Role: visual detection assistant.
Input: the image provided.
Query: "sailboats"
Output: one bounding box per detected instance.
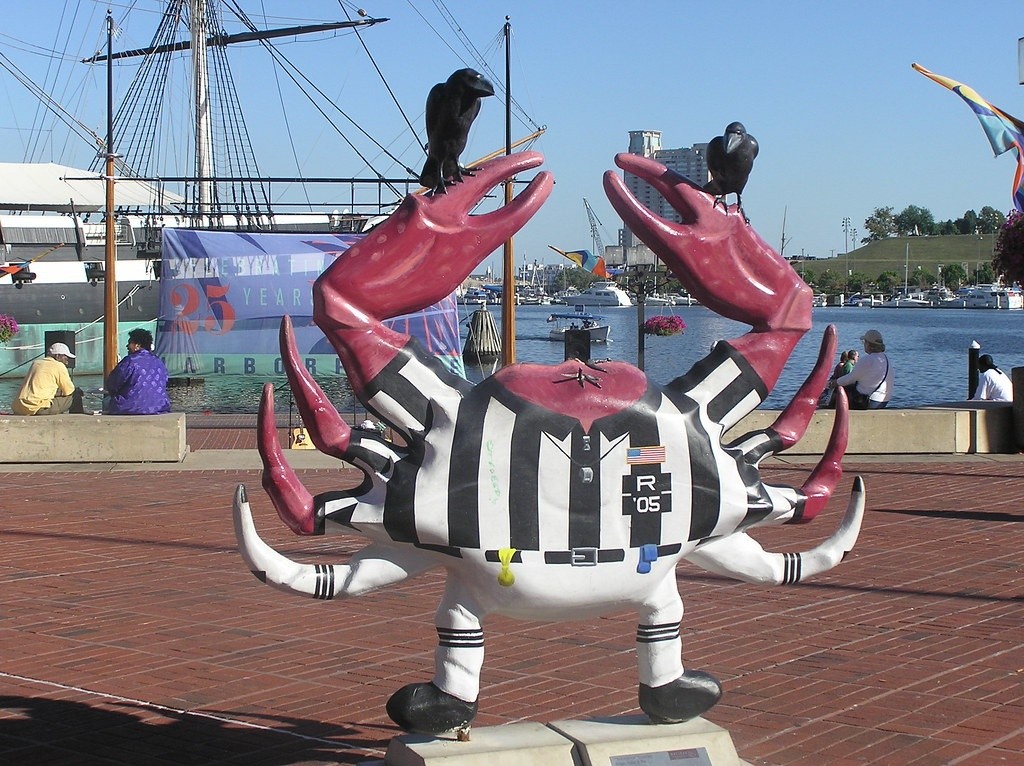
[0,0,552,387]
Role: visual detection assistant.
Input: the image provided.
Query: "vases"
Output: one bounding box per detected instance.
[656,330,680,336]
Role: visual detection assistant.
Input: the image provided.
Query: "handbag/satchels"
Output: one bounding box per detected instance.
[853,394,869,409]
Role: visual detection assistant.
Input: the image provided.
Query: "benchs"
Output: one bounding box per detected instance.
[0,412,186,462]
[721,400,1012,454]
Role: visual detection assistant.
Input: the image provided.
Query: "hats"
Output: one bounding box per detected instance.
[860,330,883,345]
[980,355,998,369]
[48,343,77,358]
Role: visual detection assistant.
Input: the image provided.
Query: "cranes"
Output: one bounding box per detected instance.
[583,197,606,265]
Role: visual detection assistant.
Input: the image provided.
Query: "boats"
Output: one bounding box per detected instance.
[457,244,704,308]
[546,311,612,343]
[812,239,1024,311]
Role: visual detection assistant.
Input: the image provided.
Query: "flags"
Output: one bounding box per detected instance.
[564,249,615,279]
[914,64,1024,214]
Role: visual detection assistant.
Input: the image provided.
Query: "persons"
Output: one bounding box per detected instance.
[570,322,576,330]
[842,349,859,374]
[103,329,171,414]
[971,355,1014,402]
[12,342,84,416]
[834,351,849,377]
[821,330,895,409]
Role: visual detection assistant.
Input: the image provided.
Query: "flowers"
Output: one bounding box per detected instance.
[0,314,18,350]
[643,315,686,339]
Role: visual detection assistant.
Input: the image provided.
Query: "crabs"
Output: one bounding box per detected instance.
[230,149,868,733]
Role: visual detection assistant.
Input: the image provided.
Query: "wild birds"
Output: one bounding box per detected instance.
[416,67,496,197]
[700,121,759,226]
